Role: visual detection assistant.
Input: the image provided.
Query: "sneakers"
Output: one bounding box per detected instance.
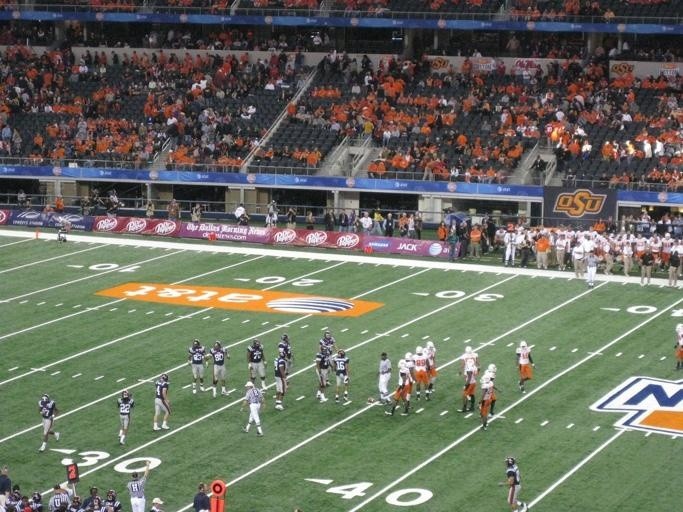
[517,380,527,394]
[316,379,349,403]
[55,432,60,441]
[242,426,264,437]
[502,263,683,290]
[192,384,230,396]
[514,503,528,511]
[259,385,284,410]
[153,425,170,430]
[456,392,494,431]
[379,391,432,417]
[38,443,46,451]
[118,433,125,445]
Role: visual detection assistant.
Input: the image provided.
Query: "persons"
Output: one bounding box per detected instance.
[674,321,683,371]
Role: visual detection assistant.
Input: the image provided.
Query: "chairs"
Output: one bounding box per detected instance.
[1,2,681,191]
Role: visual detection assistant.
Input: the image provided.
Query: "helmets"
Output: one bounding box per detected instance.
[504,456,516,466]
[253,339,260,347]
[41,394,49,404]
[399,341,434,370]
[278,334,288,358]
[10,484,117,507]
[338,350,345,358]
[321,331,332,355]
[121,390,129,400]
[480,363,498,383]
[214,340,222,351]
[465,346,475,368]
[159,373,169,383]
[520,340,527,349]
[193,339,201,348]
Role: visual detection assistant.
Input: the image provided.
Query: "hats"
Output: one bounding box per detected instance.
[151,497,165,505]
[380,352,389,358]
[244,380,255,388]
